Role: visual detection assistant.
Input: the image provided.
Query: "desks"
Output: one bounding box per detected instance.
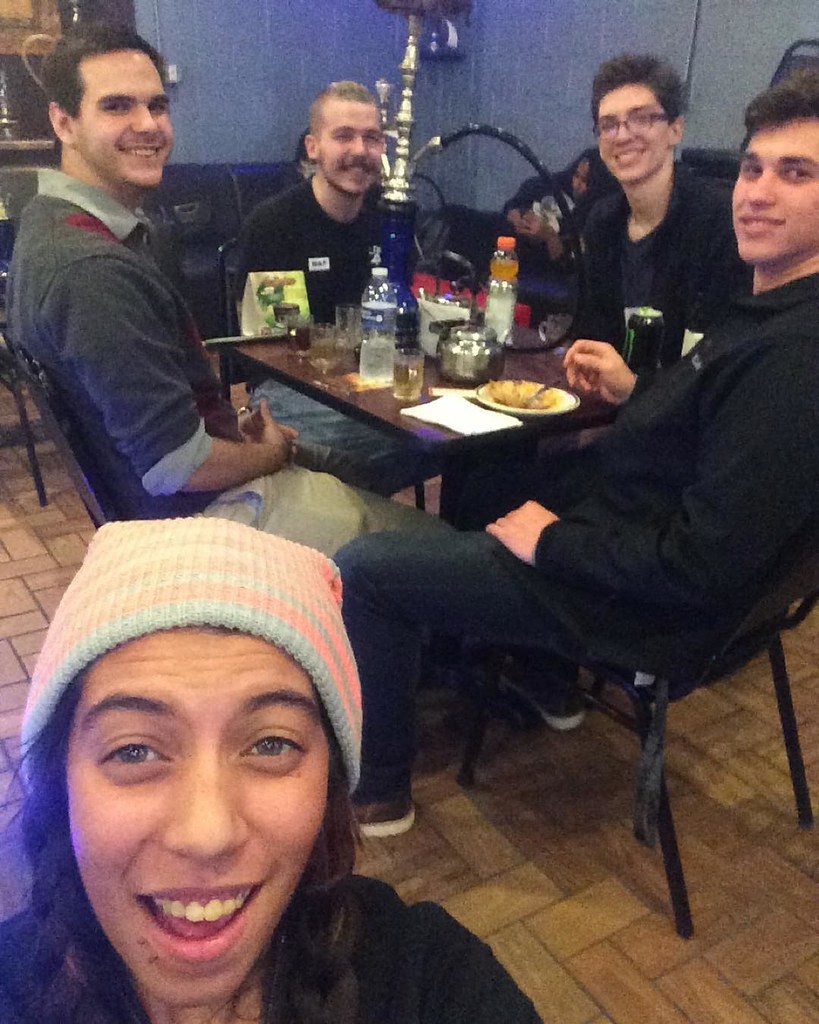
[216,327,620,736]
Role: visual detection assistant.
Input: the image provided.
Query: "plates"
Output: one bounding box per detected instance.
[475,381,581,416]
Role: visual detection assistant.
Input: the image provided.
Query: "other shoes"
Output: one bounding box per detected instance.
[503,658,581,729]
[351,771,415,839]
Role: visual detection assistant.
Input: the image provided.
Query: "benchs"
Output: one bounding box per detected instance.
[155,161,310,339]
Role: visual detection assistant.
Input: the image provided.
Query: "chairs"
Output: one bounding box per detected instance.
[0,144,818,937]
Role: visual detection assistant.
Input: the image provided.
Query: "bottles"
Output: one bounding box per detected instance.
[484,236,520,346]
[358,267,397,385]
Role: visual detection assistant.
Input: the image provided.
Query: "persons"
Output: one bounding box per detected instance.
[0,516,546,1024]
[439,51,744,528]
[330,68,819,838]
[5,18,458,553]
[238,80,440,496]
[443,148,606,274]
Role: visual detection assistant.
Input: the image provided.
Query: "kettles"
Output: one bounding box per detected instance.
[416,249,484,354]
[428,318,513,385]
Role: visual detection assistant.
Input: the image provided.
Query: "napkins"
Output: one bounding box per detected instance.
[399,394,524,437]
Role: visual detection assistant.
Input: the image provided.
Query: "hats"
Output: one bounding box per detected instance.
[17,514,365,799]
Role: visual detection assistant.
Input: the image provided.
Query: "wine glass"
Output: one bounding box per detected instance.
[308,322,340,386]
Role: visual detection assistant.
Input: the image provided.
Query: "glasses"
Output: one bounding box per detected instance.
[594,107,673,138]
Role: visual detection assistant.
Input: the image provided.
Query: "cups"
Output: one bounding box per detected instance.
[285,312,313,357]
[336,303,360,347]
[392,346,425,400]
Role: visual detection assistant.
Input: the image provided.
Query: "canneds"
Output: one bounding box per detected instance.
[621,308,665,376]
